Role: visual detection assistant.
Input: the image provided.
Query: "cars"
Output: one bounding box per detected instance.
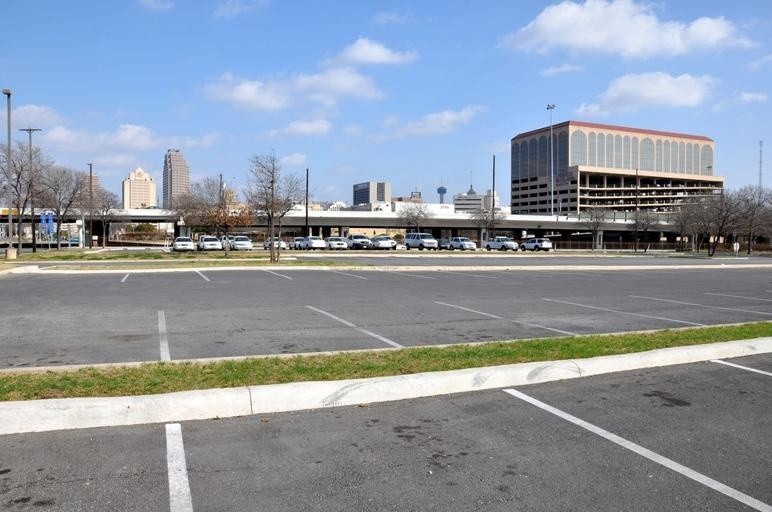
[289,237,305,250]
[197,235,223,251]
[448,237,477,251]
[436,238,452,249]
[384,237,397,250]
[172,237,194,252]
[324,237,347,250]
[262,237,286,250]
[219,235,235,250]
[303,236,326,250]
[67,237,80,245]
[370,237,392,250]
[346,234,374,250]
[233,236,253,251]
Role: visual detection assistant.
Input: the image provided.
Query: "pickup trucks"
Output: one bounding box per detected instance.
[484,235,519,252]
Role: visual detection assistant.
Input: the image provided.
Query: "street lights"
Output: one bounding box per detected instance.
[3,89,18,260]
[756,139,763,202]
[221,175,237,211]
[87,163,93,250]
[547,103,556,235]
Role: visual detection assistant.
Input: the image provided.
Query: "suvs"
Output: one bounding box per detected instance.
[404,233,439,251]
[520,237,553,252]
